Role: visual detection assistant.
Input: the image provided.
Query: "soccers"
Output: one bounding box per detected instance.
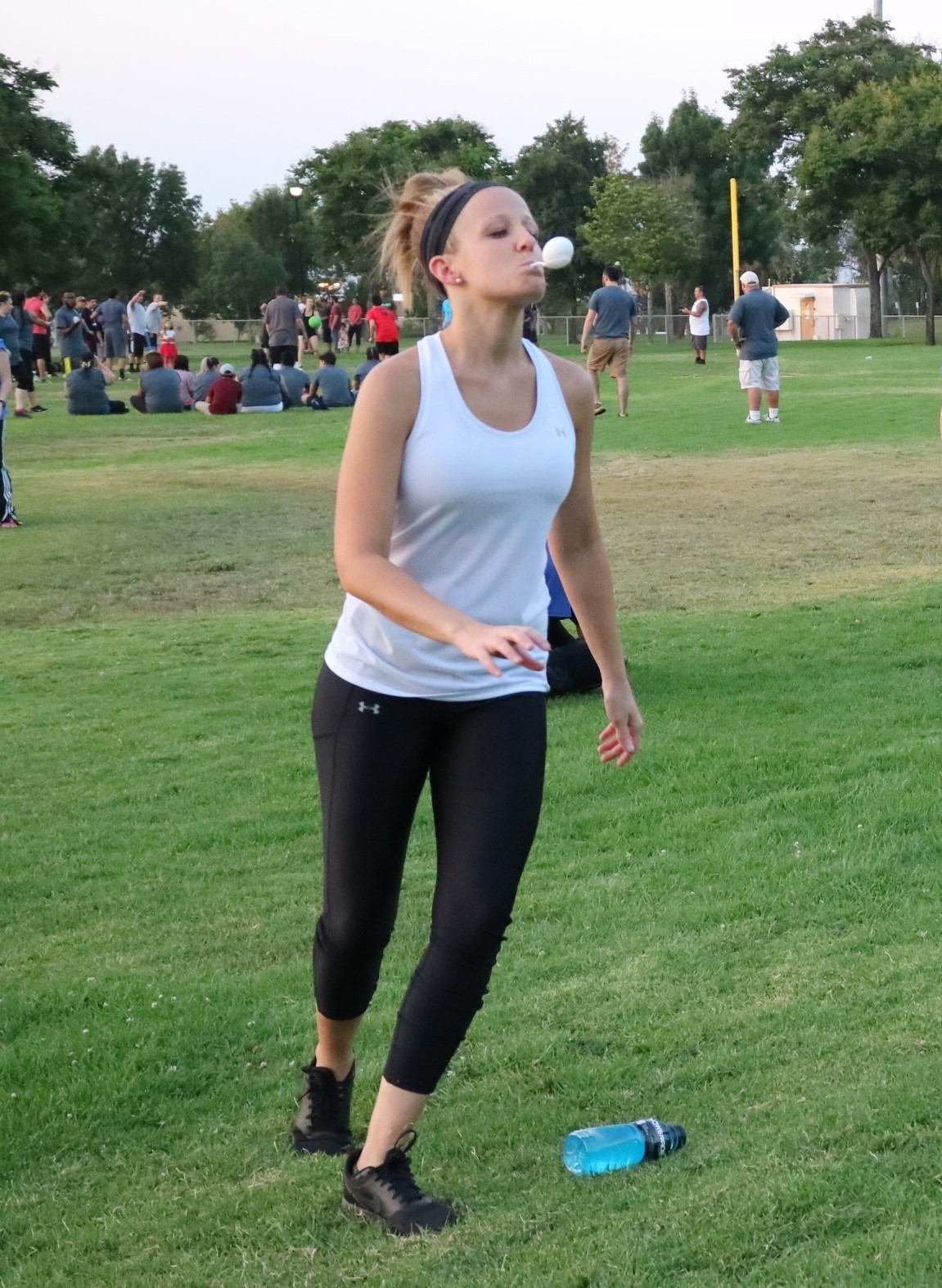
[308,316,322,328]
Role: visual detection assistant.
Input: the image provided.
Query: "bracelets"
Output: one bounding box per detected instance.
[731,337,739,342]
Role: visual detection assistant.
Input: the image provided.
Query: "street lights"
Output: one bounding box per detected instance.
[288,186,304,296]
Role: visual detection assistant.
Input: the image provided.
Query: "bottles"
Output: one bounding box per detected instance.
[562,1118,686,1176]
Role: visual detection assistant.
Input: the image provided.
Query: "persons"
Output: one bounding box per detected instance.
[288,167,646,1241]
[522,303,540,348]
[0,337,23,528]
[726,270,790,425]
[681,286,710,365]
[581,266,638,418]
[0,284,453,420]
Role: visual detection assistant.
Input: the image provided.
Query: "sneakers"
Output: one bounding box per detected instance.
[763,414,779,422]
[745,416,761,423]
[340,1129,458,1232]
[1,516,17,528]
[289,1051,356,1155]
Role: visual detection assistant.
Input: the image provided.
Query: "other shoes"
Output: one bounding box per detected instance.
[695,357,705,364]
[31,405,49,412]
[594,403,605,415]
[15,409,32,418]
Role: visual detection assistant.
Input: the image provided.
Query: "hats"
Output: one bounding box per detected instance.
[219,363,234,375]
[740,270,759,286]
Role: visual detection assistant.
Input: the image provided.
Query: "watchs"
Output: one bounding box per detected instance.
[0,400,6,409]
